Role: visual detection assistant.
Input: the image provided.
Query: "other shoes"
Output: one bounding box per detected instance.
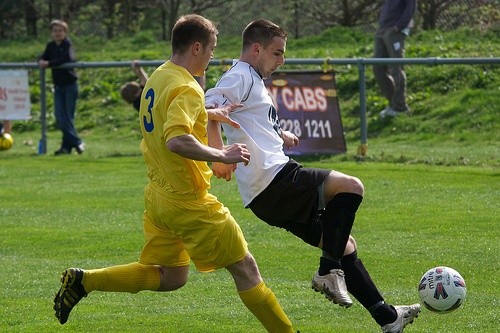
[378,107,410,118]
[55,149,71,155]
[75,142,84,154]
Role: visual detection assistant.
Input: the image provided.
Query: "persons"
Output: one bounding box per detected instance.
[121,60,151,111]
[53,14,303,333]
[203,20,424,333]
[37,19,87,155]
[372,0,415,118]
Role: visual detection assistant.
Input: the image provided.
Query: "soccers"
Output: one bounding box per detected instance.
[419,266,466,314]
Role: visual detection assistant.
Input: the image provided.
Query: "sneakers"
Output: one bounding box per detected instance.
[311,268,352,309]
[380,302,421,333]
[53,268,87,325]
